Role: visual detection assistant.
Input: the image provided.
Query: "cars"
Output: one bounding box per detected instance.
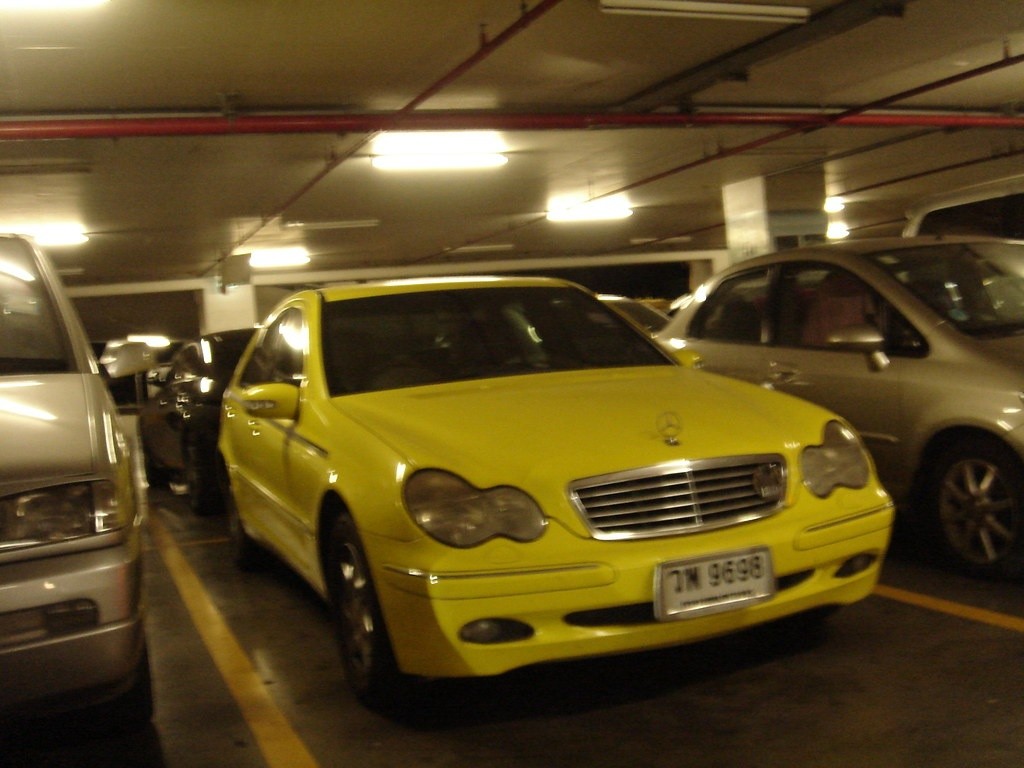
[652,233,1024,581]
[0,227,156,731]
[555,291,672,334]
[136,327,264,516]
[215,274,896,708]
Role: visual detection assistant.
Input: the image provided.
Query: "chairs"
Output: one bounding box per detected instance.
[802,272,873,348]
[333,329,395,392]
[461,320,525,375]
[881,269,954,347]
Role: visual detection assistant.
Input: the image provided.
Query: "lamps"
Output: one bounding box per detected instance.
[600,0,811,25]
